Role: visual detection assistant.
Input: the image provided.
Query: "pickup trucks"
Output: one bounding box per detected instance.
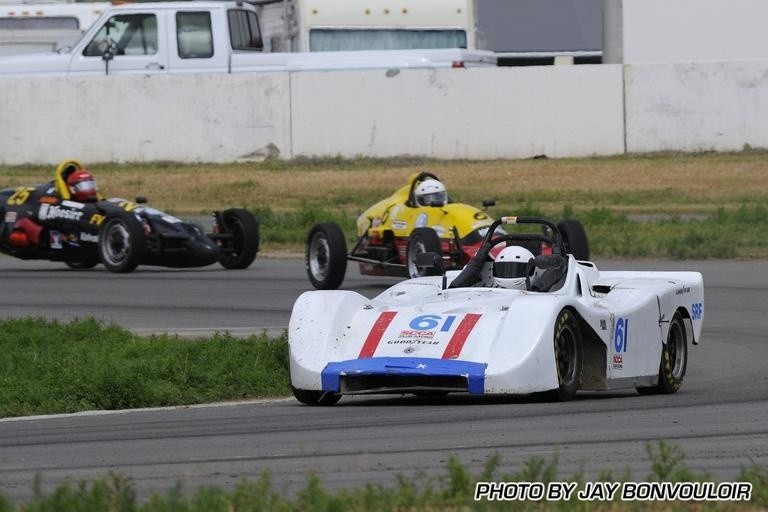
[0,3,504,79]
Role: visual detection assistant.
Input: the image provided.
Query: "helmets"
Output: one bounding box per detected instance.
[413,178,448,208]
[491,244,536,290]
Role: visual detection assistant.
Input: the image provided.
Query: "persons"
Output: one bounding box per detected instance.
[481,244,542,292]
[414,179,450,206]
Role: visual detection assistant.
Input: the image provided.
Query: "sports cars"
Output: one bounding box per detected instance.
[300,171,593,291]
[281,211,705,407]
[0,162,259,274]
